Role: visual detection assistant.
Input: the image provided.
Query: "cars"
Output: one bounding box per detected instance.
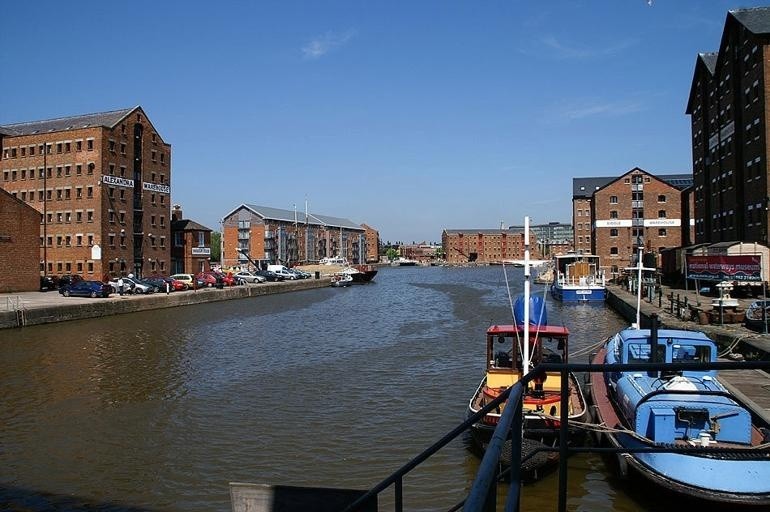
[41,265,311,298]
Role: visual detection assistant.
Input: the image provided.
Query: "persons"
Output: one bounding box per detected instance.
[127,271,134,280]
[117,276,124,296]
[128,281,135,295]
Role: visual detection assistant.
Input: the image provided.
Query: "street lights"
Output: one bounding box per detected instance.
[305,199,327,261]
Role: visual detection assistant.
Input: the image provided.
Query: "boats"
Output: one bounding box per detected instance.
[319,256,378,282]
[746,299,770,329]
[550,252,605,304]
[586,247,770,510]
[331,282,351,286]
[465,216,588,458]
